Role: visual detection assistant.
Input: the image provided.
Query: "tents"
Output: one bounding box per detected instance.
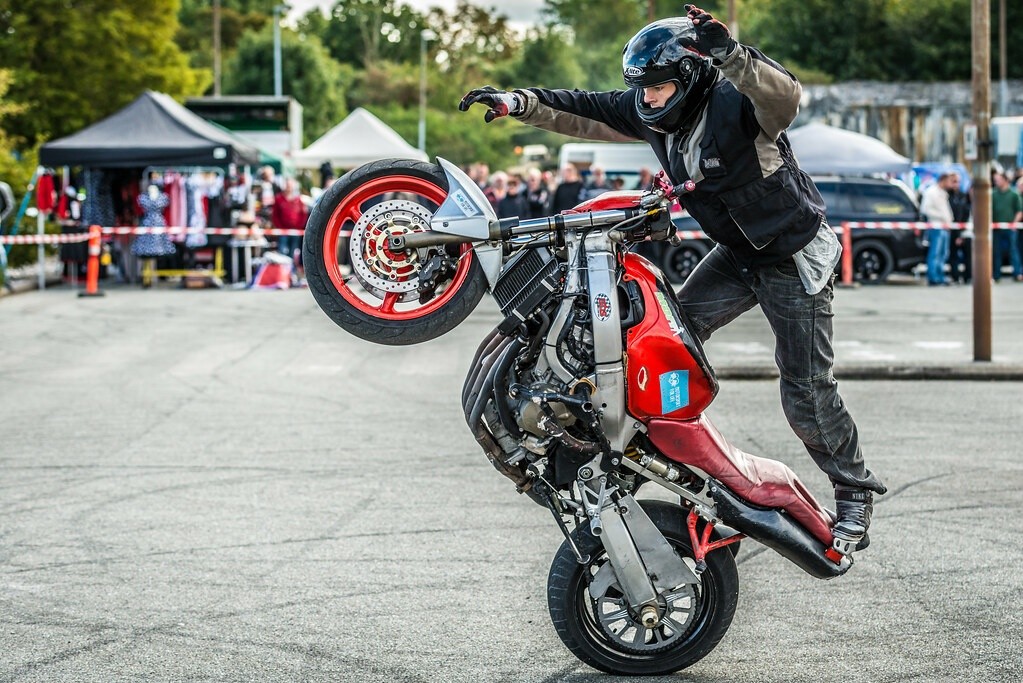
[290,105,430,170]
[37,90,294,287]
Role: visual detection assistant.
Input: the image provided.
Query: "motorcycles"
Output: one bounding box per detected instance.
[301,149,859,677]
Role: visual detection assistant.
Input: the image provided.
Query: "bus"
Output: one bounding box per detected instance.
[183,95,305,155]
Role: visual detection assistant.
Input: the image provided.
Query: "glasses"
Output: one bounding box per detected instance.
[507,181,517,186]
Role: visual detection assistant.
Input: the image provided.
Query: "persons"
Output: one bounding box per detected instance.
[946,166,1023,286]
[129,185,176,259]
[228,149,660,298]
[58,185,85,278]
[919,172,954,287]
[457,5,889,544]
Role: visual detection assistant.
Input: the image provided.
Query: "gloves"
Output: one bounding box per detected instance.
[459,85,527,124]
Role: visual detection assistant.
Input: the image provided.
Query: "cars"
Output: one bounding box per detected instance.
[630,176,931,291]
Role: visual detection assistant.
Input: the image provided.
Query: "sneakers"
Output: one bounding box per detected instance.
[830,488,873,541]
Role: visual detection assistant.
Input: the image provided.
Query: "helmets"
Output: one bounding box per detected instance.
[622,17,719,135]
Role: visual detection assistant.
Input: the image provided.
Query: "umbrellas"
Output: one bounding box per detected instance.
[770,120,912,177]
